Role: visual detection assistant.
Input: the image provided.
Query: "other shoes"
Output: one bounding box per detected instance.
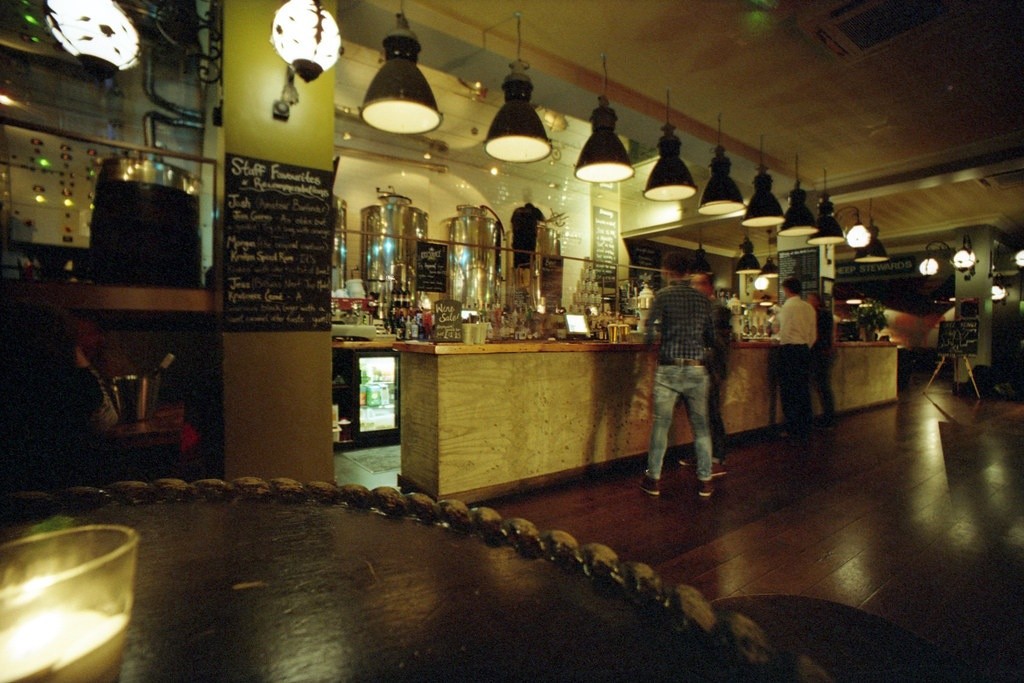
[638,475,660,494]
[699,478,715,496]
[680,455,697,465]
[710,463,728,477]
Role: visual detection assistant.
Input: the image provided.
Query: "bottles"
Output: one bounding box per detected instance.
[144,352,175,420]
[377,279,435,341]
[72,346,118,435]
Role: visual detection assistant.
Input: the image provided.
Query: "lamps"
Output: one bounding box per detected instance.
[363,15,442,135]
[45,0,139,81]
[266,0,343,120]
[484,10,552,164]
[576,53,635,184]
[642,91,1023,302]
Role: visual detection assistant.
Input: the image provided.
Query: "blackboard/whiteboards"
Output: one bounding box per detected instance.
[416,242,447,293]
[224,153,335,332]
[936,319,979,355]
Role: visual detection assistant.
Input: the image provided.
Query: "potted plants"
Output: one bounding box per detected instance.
[853,299,886,343]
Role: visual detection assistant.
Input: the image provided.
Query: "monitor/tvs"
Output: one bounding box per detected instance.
[563,312,590,337]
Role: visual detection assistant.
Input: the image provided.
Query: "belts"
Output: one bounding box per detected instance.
[659,357,704,366]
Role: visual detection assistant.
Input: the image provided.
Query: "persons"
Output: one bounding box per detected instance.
[0,312,118,485]
[779,278,817,438]
[678,272,730,475]
[807,292,837,430]
[638,252,716,496]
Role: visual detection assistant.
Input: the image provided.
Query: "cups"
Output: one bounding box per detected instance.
[607,324,618,343]
[113,374,148,423]
[631,331,648,342]
[0,525,138,683]
[462,323,473,344]
[618,324,629,342]
[474,323,488,343]
